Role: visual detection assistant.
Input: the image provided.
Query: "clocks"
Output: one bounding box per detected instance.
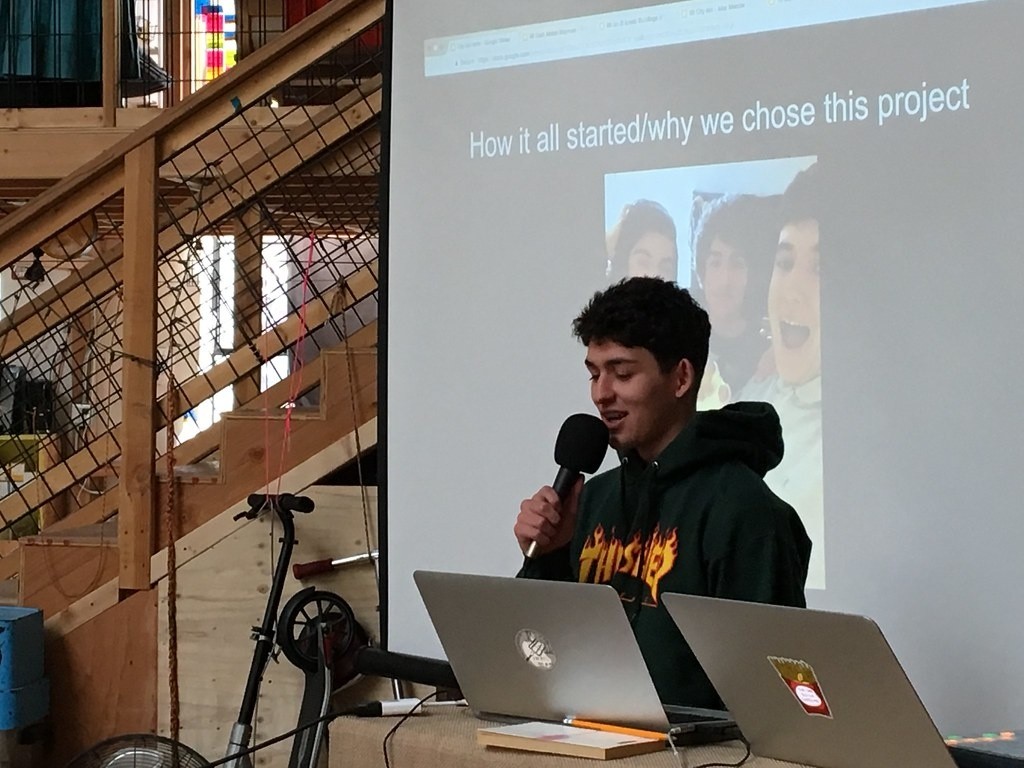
[39,210,98,258]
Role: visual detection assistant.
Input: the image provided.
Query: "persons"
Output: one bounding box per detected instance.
[607,162,822,587]
[515,277,812,712]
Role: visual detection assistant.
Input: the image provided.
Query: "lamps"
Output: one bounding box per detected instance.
[25,250,46,282]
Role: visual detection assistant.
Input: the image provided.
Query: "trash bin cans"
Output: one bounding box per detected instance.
[0,605,46,768]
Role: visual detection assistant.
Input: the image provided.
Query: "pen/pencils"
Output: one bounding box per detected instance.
[564,717,677,742]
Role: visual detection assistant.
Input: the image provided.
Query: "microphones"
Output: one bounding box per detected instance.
[524,413,609,567]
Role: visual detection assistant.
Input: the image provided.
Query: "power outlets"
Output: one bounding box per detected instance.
[71,404,90,424]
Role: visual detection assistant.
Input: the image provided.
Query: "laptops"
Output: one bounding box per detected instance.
[659,592,1024,768]
[412,571,742,746]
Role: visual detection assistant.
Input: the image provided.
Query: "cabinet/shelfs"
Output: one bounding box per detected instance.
[0,433,61,540]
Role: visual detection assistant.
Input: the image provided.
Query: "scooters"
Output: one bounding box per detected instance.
[223,491,407,767]
